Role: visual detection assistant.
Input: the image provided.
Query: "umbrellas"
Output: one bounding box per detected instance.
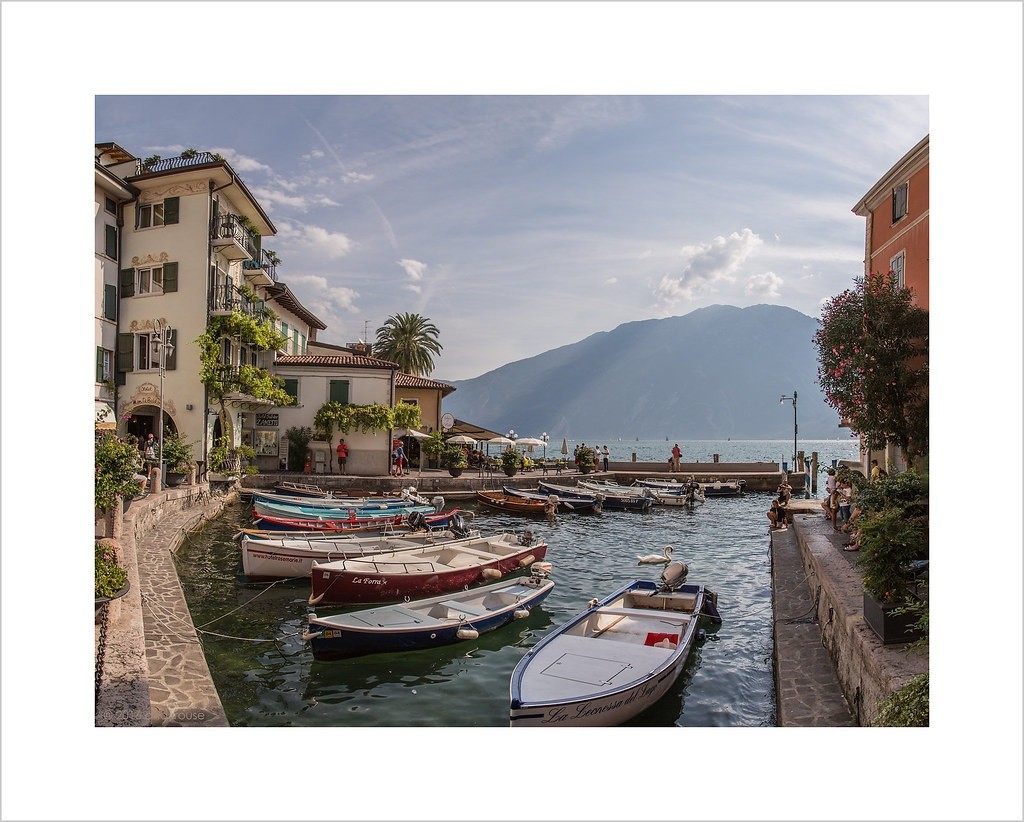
[561,438,568,466]
[486,436,516,455]
[514,438,547,470]
[445,435,478,448]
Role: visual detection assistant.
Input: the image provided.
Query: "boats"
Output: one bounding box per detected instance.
[475,488,561,516]
[240,525,482,579]
[310,525,548,605]
[537,473,750,511]
[501,485,606,514]
[233,481,473,550]
[508,559,711,728]
[308,562,556,654]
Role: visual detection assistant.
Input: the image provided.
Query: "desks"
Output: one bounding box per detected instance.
[222,222,237,237]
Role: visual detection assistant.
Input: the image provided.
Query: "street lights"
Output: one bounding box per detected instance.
[505,429,518,462]
[540,432,549,462]
[779,391,798,472]
[149,318,177,472]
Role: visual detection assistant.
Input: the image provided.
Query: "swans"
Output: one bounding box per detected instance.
[636,545,674,563]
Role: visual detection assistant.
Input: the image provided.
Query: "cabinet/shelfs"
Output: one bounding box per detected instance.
[241,427,279,456]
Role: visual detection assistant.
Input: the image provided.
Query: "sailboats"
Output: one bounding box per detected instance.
[560,438,569,455]
[527,436,536,452]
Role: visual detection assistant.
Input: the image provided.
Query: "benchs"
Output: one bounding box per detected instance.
[491,584,538,596]
[542,461,563,476]
[450,546,502,558]
[387,538,422,546]
[630,587,697,609]
[439,599,488,616]
[597,605,691,621]
[491,541,525,550]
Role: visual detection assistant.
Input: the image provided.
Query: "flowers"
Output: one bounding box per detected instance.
[95,539,129,600]
[95,407,147,509]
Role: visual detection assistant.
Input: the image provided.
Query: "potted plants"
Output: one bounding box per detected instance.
[501,447,520,477]
[154,425,202,487]
[423,427,469,477]
[574,446,597,475]
[850,494,924,644]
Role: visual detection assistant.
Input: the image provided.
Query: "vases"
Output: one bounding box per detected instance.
[102,494,134,513]
[95,578,130,623]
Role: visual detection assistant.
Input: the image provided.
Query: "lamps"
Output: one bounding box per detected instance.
[237,412,248,422]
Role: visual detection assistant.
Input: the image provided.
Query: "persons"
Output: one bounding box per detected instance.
[573,443,585,472]
[767,499,788,529]
[335,438,350,476]
[777,479,792,507]
[520,448,527,475]
[114,425,171,496]
[668,443,681,472]
[601,445,610,472]
[820,459,883,552]
[394,441,408,477]
[594,445,601,473]
